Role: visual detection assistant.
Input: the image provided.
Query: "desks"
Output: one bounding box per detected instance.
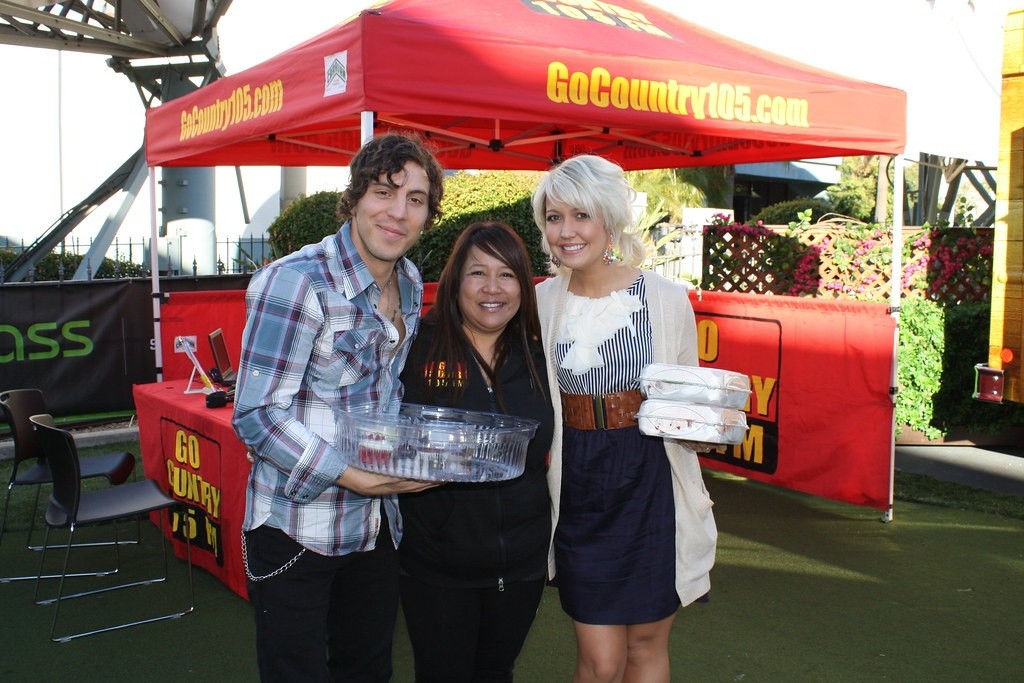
[131,377,251,601]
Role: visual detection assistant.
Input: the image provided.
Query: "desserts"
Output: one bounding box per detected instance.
[358,433,393,466]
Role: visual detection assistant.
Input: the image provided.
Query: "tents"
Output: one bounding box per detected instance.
[146,1,910,522]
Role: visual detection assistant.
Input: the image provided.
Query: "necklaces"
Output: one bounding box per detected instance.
[385,281,392,317]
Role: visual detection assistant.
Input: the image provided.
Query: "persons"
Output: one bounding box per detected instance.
[399,224,554,683]
[534,154,726,683]
[231,133,444,683]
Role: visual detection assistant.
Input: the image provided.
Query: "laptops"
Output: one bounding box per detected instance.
[207,327,238,386]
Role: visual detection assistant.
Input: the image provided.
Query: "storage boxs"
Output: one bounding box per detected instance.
[634,363,753,409]
[334,400,542,482]
[633,399,752,446]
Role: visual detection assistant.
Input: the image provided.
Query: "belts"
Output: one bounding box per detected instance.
[560,388,646,431]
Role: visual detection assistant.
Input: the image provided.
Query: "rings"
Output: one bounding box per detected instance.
[702,446,709,451]
[697,448,701,451]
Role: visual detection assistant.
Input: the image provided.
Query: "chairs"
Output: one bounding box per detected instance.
[0,388,141,584]
[28,414,195,643]
